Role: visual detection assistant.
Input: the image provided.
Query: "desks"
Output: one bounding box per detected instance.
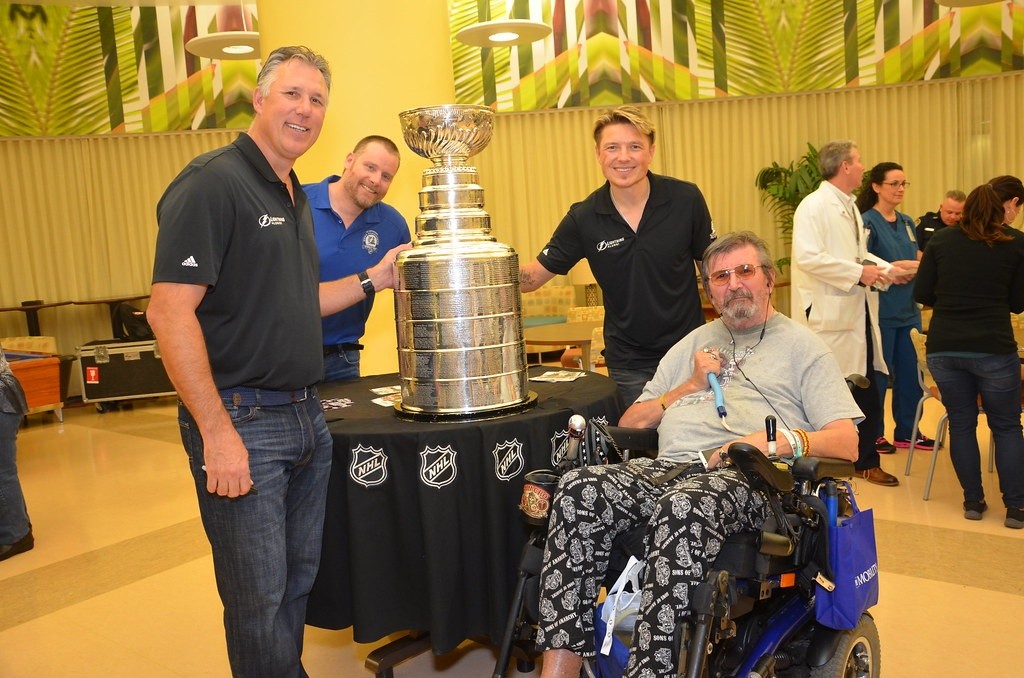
[73,294,150,339]
[524,323,603,372]
[524,317,567,328]
[305,367,622,678]
[0,301,73,336]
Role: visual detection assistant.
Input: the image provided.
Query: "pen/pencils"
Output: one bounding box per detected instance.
[202,466,258,495]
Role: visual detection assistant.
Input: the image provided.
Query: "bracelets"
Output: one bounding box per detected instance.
[356,271,376,298]
[659,391,666,412]
[776,427,809,457]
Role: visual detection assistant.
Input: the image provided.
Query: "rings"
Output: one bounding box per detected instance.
[709,353,717,360]
[704,346,714,353]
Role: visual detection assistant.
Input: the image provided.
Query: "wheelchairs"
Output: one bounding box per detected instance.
[492,412,882,678]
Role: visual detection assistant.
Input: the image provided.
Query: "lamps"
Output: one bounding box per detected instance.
[455,0,552,49]
[184,0,262,61]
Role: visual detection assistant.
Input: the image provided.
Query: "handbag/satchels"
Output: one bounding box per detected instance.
[590,554,646,678]
[814,477,880,631]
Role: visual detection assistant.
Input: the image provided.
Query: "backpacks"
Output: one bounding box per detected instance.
[112,302,156,341]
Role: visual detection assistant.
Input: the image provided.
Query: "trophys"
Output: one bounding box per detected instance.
[392,104,529,415]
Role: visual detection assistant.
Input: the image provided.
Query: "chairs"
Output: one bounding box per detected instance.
[520,283,608,374]
[905,327,994,501]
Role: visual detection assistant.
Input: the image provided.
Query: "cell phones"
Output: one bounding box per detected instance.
[857,259,877,288]
[699,445,728,472]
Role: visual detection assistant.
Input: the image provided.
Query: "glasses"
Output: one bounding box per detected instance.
[704,263,764,286]
[878,181,910,190]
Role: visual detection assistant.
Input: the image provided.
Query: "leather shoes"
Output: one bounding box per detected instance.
[0,529,34,561]
[853,466,899,486]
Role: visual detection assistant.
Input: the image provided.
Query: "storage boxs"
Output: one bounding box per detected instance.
[78,338,178,413]
[6,357,64,425]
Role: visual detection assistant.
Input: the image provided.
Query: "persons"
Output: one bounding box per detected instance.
[533,232,866,678]
[916,175,1024,529]
[299,134,411,381]
[517,106,717,414]
[855,162,944,453]
[789,140,915,487]
[914,190,966,252]
[146,43,412,678]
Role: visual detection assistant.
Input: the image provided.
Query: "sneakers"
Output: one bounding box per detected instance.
[875,436,896,453]
[964,498,988,520]
[894,432,942,451]
[1005,507,1024,529]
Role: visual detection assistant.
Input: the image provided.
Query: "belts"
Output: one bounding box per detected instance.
[215,387,312,407]
[321,342,364,357]
